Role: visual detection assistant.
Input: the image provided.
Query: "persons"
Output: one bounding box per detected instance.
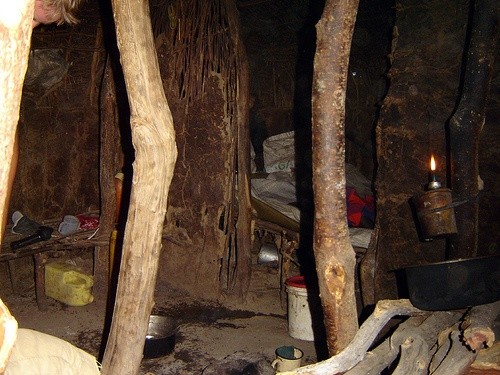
[31,0,79,31]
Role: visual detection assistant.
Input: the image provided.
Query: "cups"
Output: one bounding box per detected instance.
[58,214,80,235]
[271,344,305,374]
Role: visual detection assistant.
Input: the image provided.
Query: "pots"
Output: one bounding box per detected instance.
[141,313,191,359]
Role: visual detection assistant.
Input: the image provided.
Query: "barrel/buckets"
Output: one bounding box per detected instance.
[43,260,96,309]
[284,275,328,343]
